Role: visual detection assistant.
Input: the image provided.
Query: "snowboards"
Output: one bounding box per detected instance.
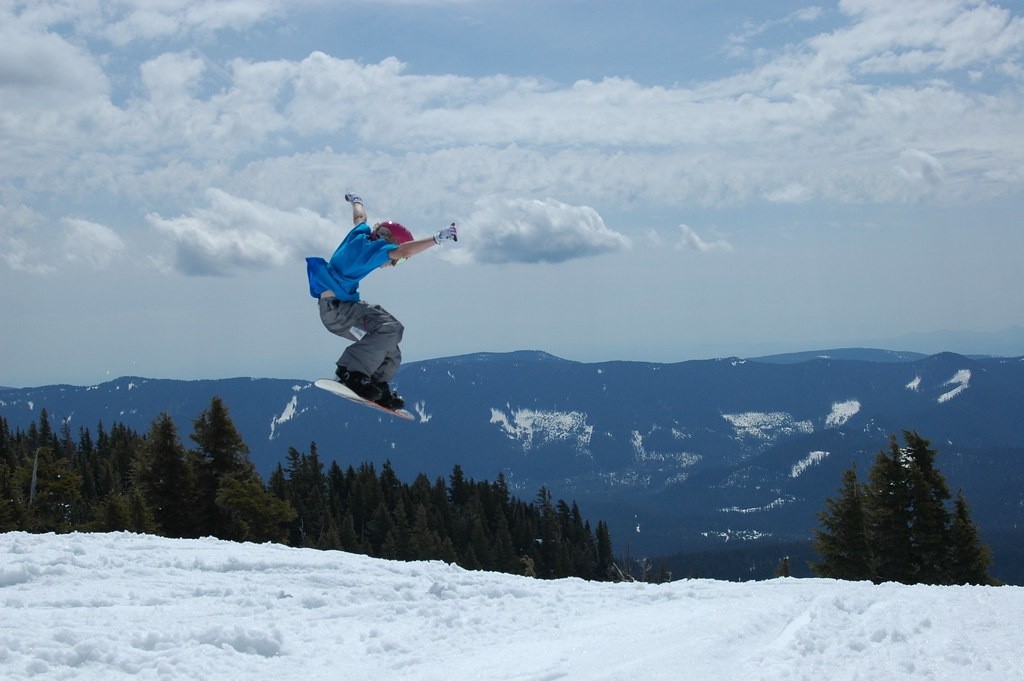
[313,378,416,422]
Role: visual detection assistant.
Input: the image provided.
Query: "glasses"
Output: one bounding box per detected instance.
[394,257,408,269]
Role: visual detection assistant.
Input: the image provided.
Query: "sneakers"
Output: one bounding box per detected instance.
[335,362,382,402]
[371,375,405,410]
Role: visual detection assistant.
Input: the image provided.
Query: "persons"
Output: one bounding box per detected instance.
[306,192,457,408]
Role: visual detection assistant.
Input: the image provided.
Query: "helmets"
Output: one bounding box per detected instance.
[372,222,415,245]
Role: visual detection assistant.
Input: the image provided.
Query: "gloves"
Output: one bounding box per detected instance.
[433,222,459,244]
[344,191,364,205]
